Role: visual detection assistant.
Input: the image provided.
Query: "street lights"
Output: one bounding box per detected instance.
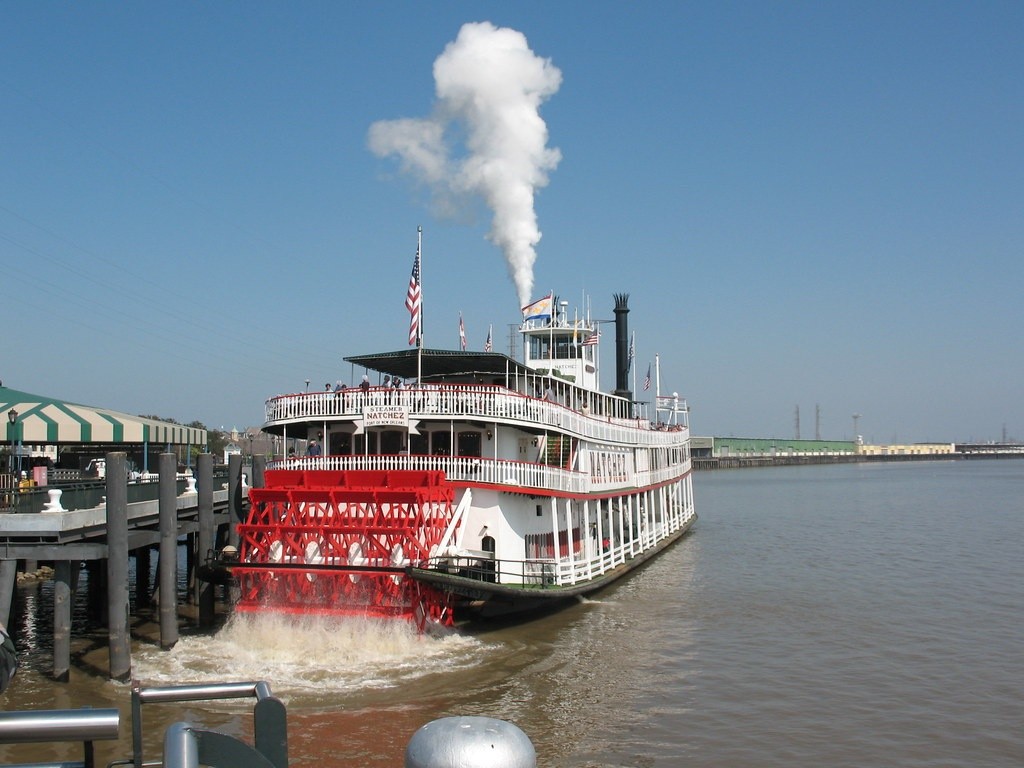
[7,408,19,513]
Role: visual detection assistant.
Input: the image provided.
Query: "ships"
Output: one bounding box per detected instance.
[198,223,700,644]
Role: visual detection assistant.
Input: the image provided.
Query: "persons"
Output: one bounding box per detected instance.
[286,447,299,460]
[274,373,407,415]
[302,438,321,458]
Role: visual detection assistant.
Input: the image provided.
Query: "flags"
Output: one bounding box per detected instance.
[405,236,424,346]
[484,324,492,353]
[582,327,597,346]
[521,293,551,324]
[457,309,466,352]
[642,364,650,391]
[628,332,634,374]
